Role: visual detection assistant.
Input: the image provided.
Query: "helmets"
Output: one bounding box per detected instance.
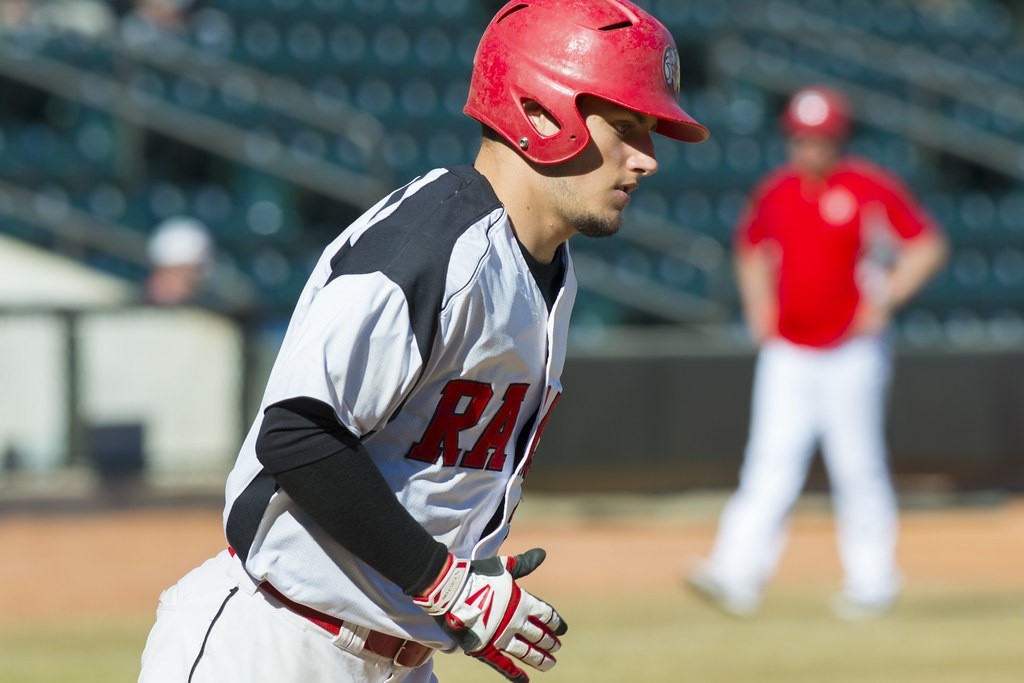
[463,0,709,166]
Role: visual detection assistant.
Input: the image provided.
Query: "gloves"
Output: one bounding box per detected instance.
[413,547,568,683]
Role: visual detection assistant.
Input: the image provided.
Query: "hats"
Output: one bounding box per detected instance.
[782,86,853,140]
[146,219,211,265]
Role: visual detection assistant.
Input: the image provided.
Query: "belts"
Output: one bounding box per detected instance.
[229,546,432,669]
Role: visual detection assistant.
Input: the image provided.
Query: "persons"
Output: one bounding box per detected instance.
[145,217,262,338]
[677,88,946,621]
[138,0,710,683]
